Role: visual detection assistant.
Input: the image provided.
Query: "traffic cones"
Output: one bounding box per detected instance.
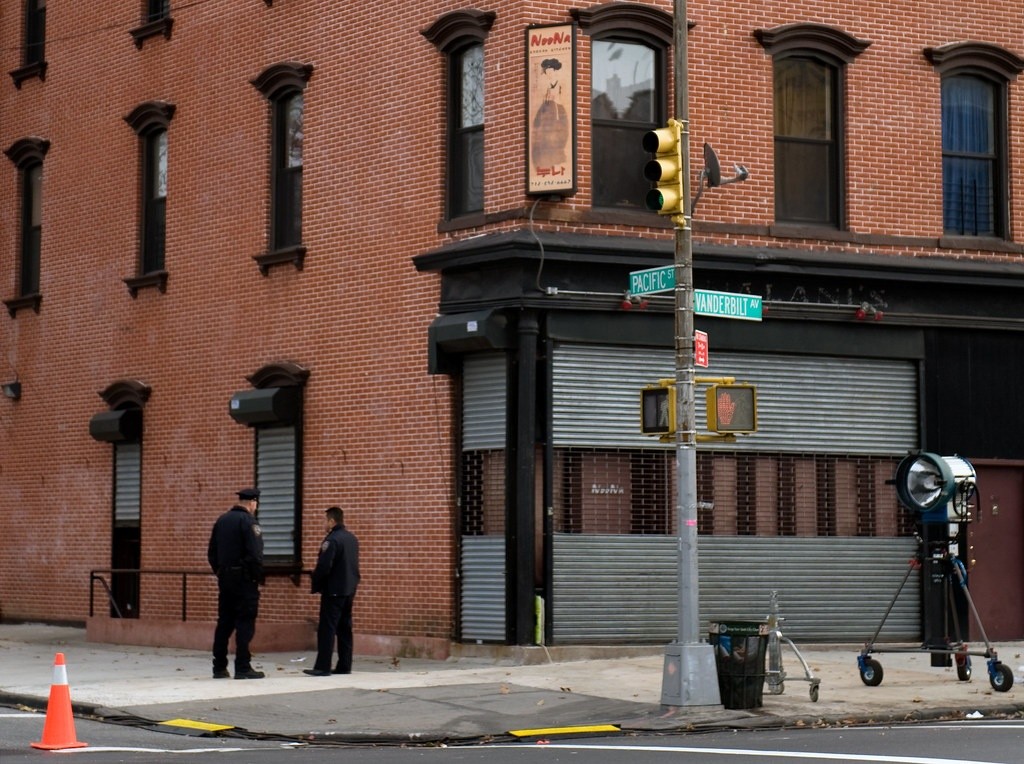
[30,652,88,750]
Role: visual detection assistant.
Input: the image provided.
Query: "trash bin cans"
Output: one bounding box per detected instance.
[708,618,771,710]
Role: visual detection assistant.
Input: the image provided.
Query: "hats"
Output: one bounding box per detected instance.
[236,489,261,500]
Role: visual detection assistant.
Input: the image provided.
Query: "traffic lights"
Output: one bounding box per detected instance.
[707,384,757,434]
[642,118,684,216]
[641,385,677,436]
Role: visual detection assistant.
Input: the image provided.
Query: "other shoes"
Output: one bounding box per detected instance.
[234,668,264,679]
[213,669,230,678]
[331,669,351,673]
[303,669,331,676]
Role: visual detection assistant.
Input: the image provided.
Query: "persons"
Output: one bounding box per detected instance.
[303,506,362,676]
[208,488,267,679]
